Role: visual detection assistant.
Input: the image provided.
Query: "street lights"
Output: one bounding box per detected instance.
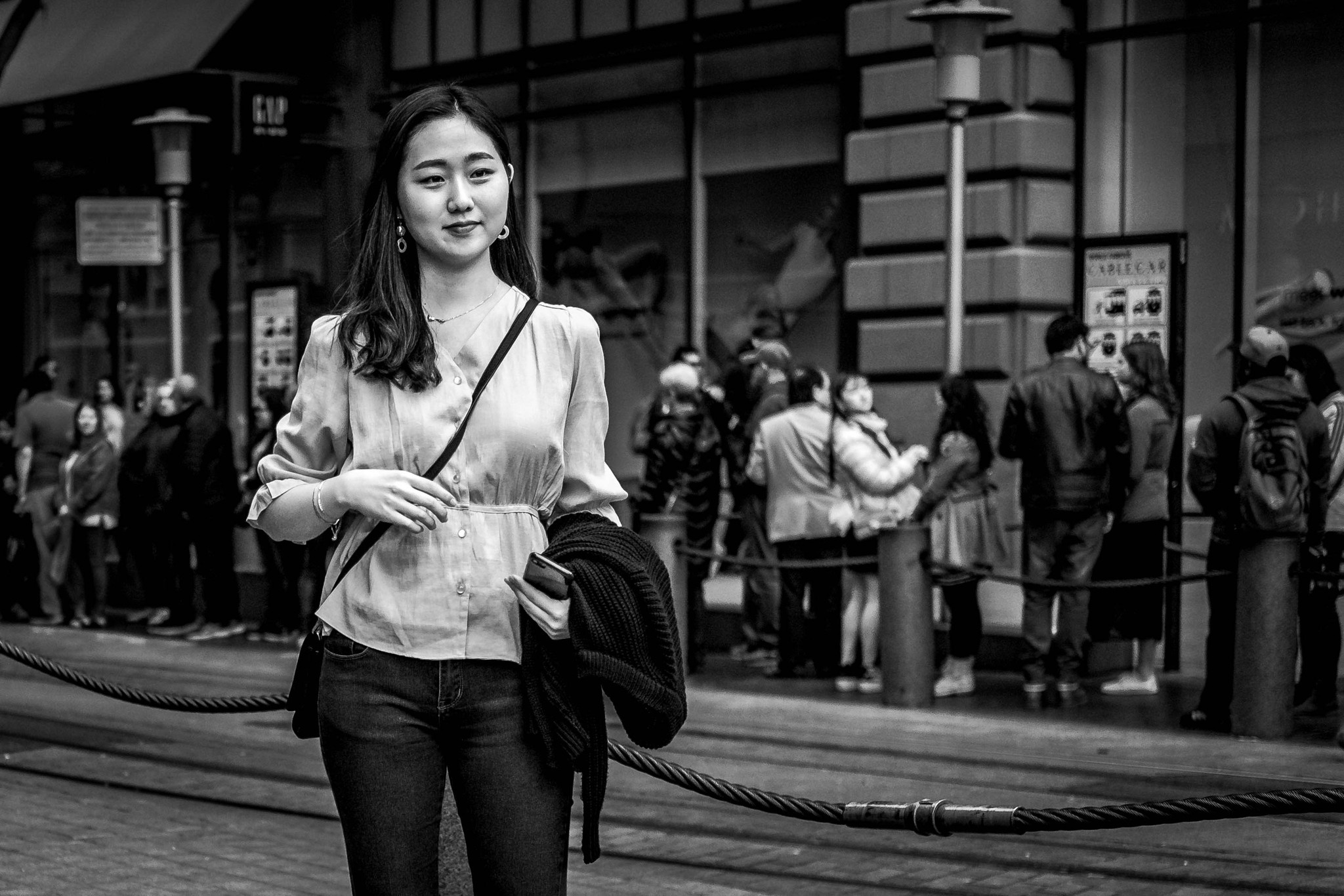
[132,105,210,392]
[903,0,1012,394]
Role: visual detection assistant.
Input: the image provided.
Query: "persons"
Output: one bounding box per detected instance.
[0,349,339,649]
[246,84,629,895]
[618,312,1343,727]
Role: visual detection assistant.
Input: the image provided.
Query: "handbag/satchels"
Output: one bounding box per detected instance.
[282,633,326,740]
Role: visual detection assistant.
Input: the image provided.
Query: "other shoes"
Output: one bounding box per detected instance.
[1024,671,1081,693]
[33,605,248,641]
[933,655,978,695]
[1099,673,1159,695]
[246,627,302,643]
[835,664,884,694]
[1178,710,1230,731]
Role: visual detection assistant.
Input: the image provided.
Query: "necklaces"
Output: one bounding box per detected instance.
[422,274,500,323]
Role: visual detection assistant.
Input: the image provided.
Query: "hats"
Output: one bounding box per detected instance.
[1239,327,1289,366]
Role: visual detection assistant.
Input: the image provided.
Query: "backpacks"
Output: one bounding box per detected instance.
[1223,392,1314,538]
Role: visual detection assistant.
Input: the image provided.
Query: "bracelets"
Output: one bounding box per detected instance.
[313,480,343,540]
